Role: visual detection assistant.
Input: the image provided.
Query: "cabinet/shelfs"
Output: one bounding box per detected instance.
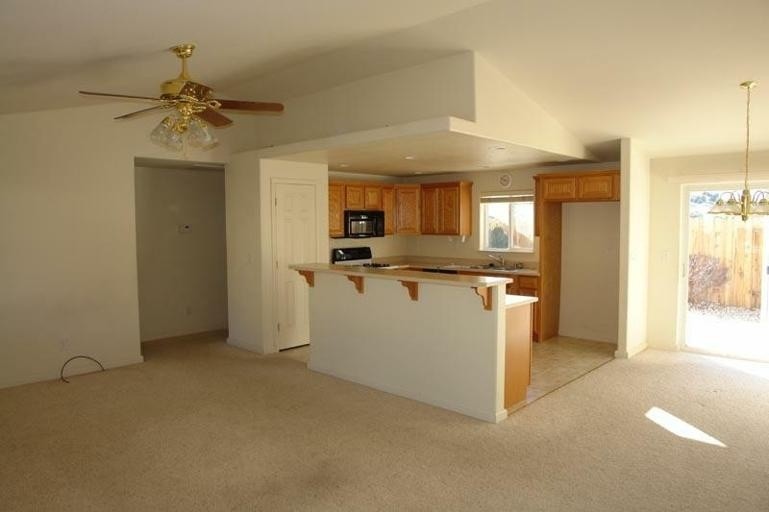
[537,170,620,202]
[328,182,345,238]
[500,276,540,343]
[345,183,382,212]
[383,184,397,236]
[398,184,423,236]
[422,179,474,235]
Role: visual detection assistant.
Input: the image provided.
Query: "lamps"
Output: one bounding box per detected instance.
[147,96,222,153]
[707,80,769,221]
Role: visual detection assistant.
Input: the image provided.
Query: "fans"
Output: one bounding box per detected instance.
[75,44,288,129]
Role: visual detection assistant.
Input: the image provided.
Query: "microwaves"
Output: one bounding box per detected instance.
[343,209,385,238]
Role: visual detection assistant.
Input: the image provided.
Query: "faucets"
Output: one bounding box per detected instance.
[488,254,505,265]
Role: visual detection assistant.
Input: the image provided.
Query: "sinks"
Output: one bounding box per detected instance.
[439,263,494,273]
[492,267,518,274]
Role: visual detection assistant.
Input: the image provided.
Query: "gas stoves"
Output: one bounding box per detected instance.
[359,263,400,270]
